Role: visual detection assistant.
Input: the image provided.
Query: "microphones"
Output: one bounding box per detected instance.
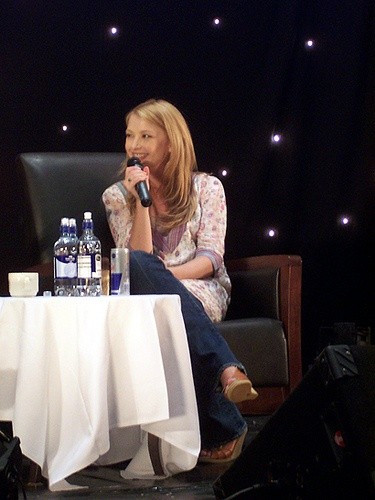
[127,156,152,207]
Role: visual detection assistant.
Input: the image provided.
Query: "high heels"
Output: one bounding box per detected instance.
[199,424,247,463]
[220,365,258,403]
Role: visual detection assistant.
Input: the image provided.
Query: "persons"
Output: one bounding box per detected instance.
[101,99,259,464]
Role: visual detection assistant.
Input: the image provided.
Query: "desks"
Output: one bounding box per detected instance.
[0,295,201,492]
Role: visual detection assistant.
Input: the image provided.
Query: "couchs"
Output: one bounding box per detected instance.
[16,151,303,416]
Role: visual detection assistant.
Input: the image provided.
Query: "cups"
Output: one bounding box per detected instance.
[7,272,39,297]
[101,256,109,296]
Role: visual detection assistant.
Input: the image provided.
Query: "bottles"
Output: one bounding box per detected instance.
[78,212,102,297]
[53,217,78,297]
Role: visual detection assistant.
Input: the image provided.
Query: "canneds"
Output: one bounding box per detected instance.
[110,248,130,296]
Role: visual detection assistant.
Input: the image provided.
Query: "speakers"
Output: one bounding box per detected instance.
[212,345,375,500]
